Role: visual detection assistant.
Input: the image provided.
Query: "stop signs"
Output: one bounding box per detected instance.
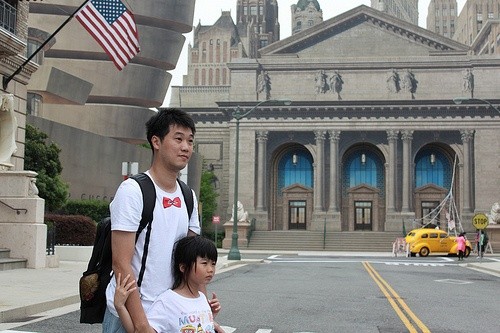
[472,213,489,230]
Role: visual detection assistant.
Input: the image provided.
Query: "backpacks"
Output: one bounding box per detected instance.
[79,174,194,324]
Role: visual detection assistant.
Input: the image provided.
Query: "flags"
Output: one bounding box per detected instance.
[75,0,141,71]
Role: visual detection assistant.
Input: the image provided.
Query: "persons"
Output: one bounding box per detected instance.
[386,69,400,92]
[329,72,341,92]
[475,228,486,256]
[103,109,226,333]
[258,70,271,93]
[315,71,327,94]
[461,70,473,89]
[113,236,222,333]
[403,69,415,92]
[457,233,466,260]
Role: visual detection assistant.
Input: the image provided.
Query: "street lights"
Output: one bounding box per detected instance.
[225,99,292,264]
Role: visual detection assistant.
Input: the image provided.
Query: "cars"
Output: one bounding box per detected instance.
[404,229,473,258]
